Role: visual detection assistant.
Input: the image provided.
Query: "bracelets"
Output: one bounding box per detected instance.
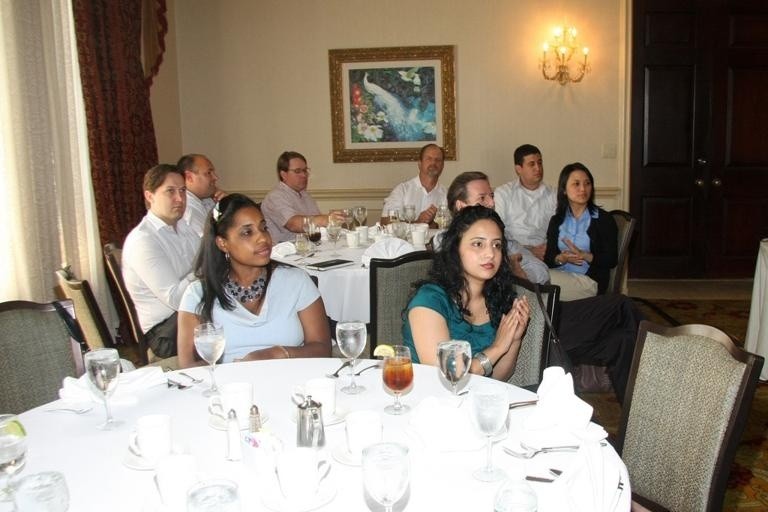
[474,352,492,377]
[276,345,289,359]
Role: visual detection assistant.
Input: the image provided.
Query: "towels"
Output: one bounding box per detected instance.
[431,226,448,253]
[318,226,350,240]
[388,223,414,234]
[270,241,297,260]
[521,365,602,469]
[58,363,169,411]
[362,237,415,268]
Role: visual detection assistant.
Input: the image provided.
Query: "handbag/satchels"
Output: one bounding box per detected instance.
[573,365,612,392]
[549,339,573,379]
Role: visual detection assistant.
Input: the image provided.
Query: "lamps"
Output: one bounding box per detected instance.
[540,21,590,84]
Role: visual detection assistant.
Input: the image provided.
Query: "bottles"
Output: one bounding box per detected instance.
[223,409,243,461]
[249,402,261,432]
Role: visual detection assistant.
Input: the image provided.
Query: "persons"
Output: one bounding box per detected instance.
[176,192,332,368]
[400,203,531,383]
[260,151,344,246]
[492,144,558,261]
[177,154,228,237]
[121,163,202,359]
[433,171,551,286]
[543,162,618,302]
[380,144,454,229]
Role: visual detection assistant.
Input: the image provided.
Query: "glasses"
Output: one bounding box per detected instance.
[212,195,252,225]
[285,169,310,174]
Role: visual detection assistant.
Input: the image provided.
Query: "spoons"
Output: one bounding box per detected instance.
[327,361,348,380]
[502,438,609,462]
[169,366,206,384]
[350,363,379,378]
[45,407,94,415]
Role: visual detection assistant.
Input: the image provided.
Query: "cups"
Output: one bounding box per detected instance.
[345,225,370,247]
[438,204,452,229]
[410,222,432,249]
[13,469,69,511]
[493,482,539,512]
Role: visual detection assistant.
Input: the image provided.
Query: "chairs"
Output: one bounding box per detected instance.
[368,251,440,360]
[0,242,163,421]
[556,209,638,305]
[614,320,765,511]
[508,273,561,391]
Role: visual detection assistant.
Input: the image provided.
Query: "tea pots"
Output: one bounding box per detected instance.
[290,390,326,450]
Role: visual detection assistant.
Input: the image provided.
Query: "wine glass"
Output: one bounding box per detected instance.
[297,210,341,262]
[0,412,29,501]
[380,344,414,418]
[360,440,415,512]
[81,346,128,432]
[341,204,368,230]
[191,320,230,398]
[383,202,416,239]
[334,316,369,397]
[468,380,512,483]
[434,339,473,419]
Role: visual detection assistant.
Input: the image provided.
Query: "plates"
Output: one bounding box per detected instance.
[209,409,270,432]
[289,403,351,426]
[120,436,371,512]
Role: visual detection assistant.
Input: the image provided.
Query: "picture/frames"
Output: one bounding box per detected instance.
[328,44,457,164]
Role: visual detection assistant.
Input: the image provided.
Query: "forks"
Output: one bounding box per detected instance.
[165,377,189,393]
